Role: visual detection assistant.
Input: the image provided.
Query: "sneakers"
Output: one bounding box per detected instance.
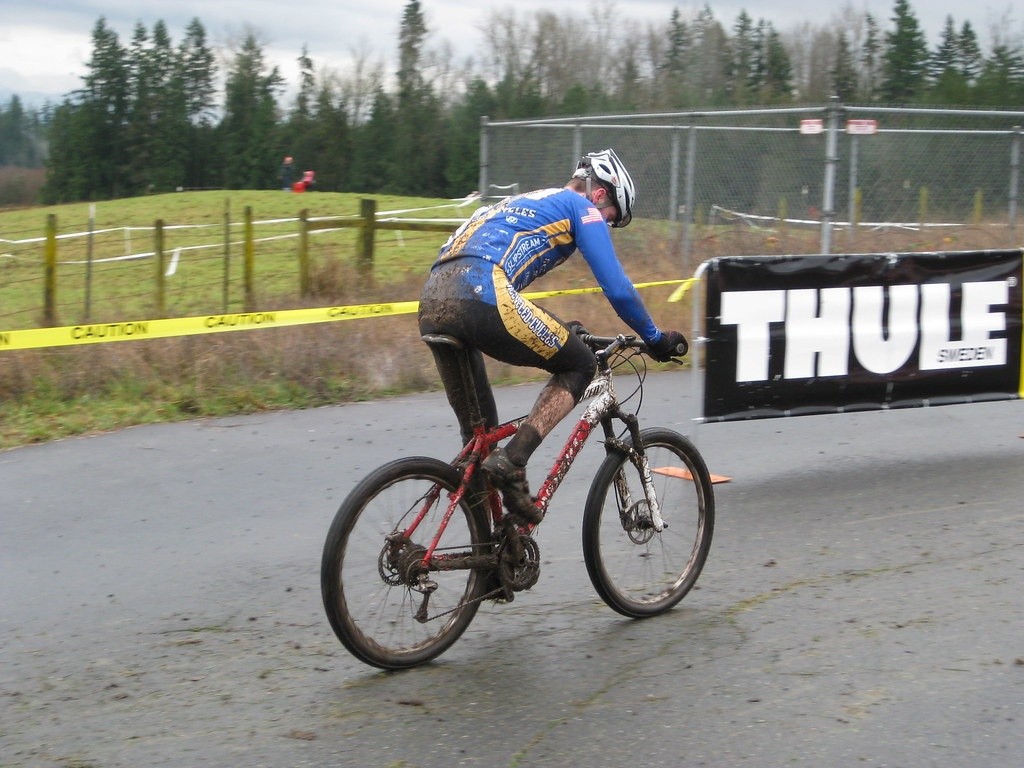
[479,574,513,601]
[480,448,543,524]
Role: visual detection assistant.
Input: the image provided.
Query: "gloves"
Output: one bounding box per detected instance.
[649,330,689,359]
[566,320,587,340]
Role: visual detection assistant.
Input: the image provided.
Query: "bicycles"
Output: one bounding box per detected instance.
[320,331,716,671]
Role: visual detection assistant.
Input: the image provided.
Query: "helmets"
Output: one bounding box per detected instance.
[573,146,635,228]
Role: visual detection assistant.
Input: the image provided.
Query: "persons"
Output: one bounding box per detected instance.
[416,148,688,601]
[276,155,303,190]
